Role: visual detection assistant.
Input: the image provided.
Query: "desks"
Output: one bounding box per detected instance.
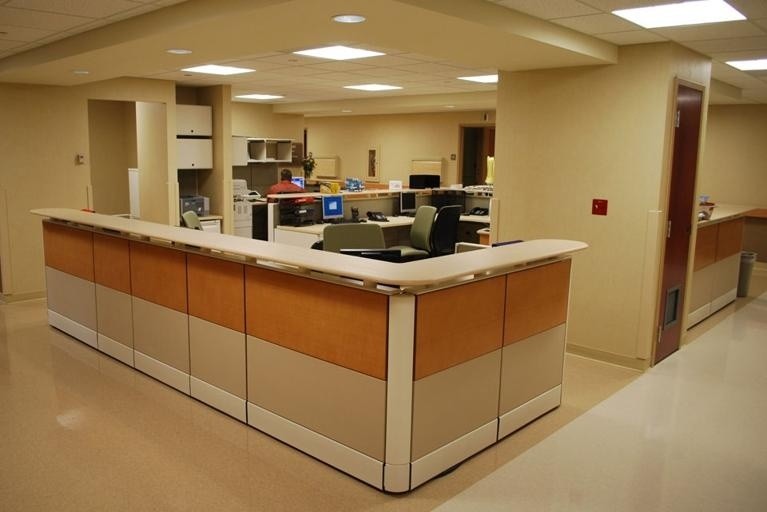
[274,208,490,247]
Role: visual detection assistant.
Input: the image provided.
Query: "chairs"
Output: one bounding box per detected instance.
[322,204,462,262]
[182,211,204,231]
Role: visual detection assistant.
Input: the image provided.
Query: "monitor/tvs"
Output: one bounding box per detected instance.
[399,191,417,216]
[339,248,401,261]
[290,176,304,189]
[321,194,344,224]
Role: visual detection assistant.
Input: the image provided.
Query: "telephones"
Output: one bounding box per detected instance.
[367,211,389,222]
[470,207,488,215]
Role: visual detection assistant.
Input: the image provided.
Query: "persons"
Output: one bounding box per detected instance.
[262,168,309,199]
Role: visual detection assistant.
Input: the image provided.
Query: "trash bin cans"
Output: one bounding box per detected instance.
[737,251,758,296]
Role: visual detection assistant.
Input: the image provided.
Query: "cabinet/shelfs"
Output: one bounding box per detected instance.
[175,104,212,170]
[233,137,292,166]
